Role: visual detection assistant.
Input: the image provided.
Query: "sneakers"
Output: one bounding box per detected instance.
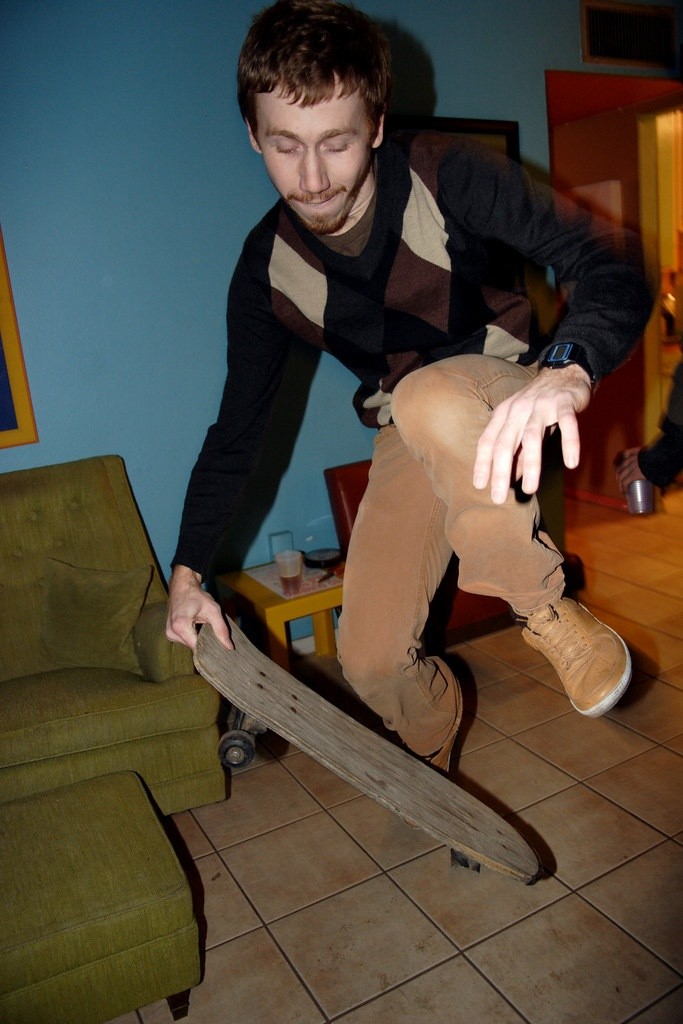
[516,596,631,718]
[401,732,457,779]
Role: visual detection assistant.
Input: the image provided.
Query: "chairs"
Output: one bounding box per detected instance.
[323,459,584,652]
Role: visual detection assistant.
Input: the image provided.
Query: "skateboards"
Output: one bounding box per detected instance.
[192,612,542,885]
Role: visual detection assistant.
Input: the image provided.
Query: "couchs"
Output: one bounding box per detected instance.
[0,455,228,818]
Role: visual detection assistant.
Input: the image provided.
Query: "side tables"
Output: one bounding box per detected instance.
[214,560,344,674]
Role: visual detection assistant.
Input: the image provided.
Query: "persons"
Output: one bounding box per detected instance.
[615,362,682,496]
[164,1,663,776]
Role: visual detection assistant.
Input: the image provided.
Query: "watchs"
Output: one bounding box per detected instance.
[541,342,596,390]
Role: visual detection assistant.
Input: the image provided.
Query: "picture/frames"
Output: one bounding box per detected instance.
[0,229,41,450]
[387,115,521,174]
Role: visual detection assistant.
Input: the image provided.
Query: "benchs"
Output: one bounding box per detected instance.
[0,769,201,1024]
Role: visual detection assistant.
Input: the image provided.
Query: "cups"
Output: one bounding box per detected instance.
[626,480,653,516]
[275,550,302,597]
